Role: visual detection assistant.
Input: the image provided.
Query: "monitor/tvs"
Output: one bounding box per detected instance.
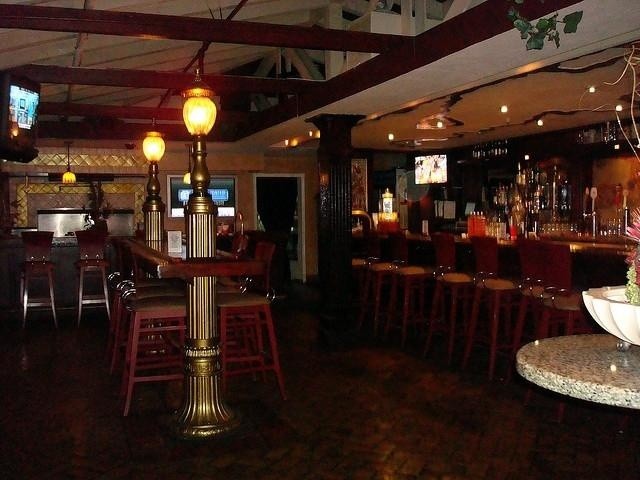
[0,71,41,162]
[413,153,449,185]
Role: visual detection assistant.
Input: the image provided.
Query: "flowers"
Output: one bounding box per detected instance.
[616,204,640,303]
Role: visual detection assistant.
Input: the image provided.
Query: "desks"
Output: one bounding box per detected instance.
[513,332,640,415]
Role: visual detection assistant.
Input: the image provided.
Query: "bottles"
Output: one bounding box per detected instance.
[600,120,617,142]
[473,138,509,158]
[467,161,577,240]
[599,205,630,238]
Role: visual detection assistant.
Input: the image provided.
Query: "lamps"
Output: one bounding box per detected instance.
[179,62,217,215]
[142,116,167,210]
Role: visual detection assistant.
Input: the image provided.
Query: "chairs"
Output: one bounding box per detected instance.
[105,235,288,418]
[516,239,591,422]
[349,228,434,351]
[461,234,518,376]
[421,231,470,367]
[17,229,117,327]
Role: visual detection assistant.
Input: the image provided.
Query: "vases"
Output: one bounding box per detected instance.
[581,283,640,358]
